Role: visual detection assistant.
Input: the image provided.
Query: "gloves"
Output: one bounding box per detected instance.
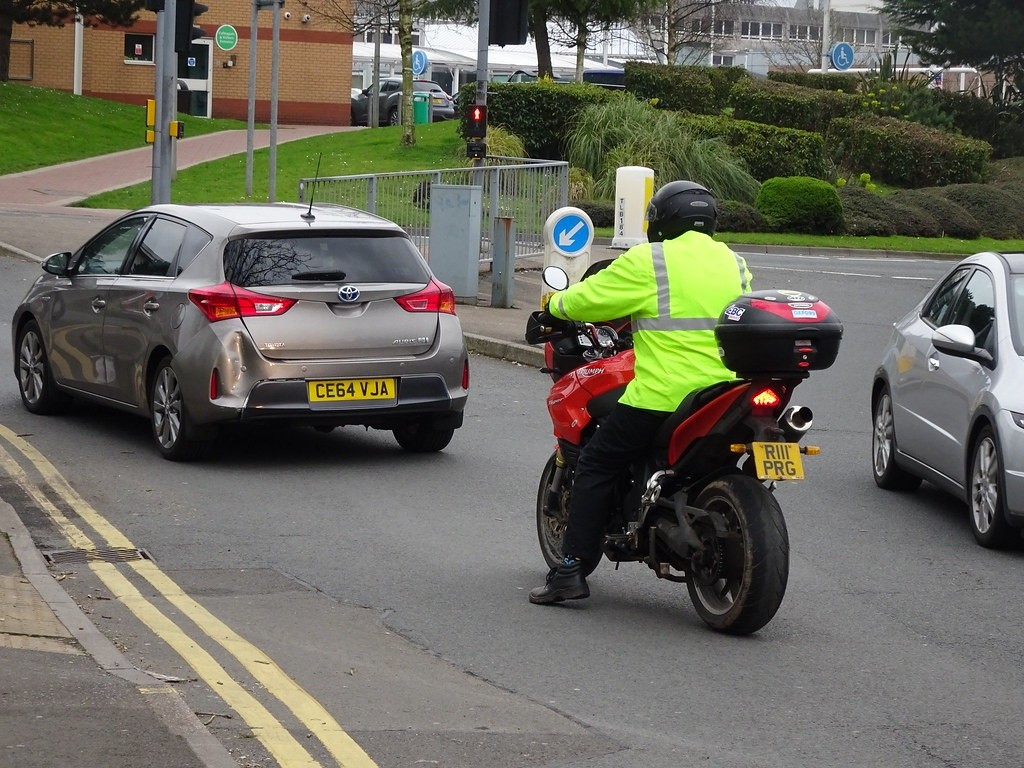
[535,303,570,341]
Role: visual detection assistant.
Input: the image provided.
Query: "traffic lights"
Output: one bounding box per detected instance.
[171,120,184,138]
[145,99,155,128]
[467,105,486,136]
[146,129,153,143]
[187,1,207,42]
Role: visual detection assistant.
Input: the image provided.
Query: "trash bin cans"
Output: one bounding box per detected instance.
[413,92,428,125]
[176,80,192,115]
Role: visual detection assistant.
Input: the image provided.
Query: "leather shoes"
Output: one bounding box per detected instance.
[528,559,590,604]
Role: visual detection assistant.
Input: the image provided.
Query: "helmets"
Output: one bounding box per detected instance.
[643,179,718,243]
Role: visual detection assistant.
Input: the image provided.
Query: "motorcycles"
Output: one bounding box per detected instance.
[525,258,844,635]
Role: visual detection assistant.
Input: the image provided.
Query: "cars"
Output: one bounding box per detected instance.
[871,252,1024,549]
[12,201,469,462]
[351,78,455,127]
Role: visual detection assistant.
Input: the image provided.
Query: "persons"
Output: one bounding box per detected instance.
[529,181,754,604]
